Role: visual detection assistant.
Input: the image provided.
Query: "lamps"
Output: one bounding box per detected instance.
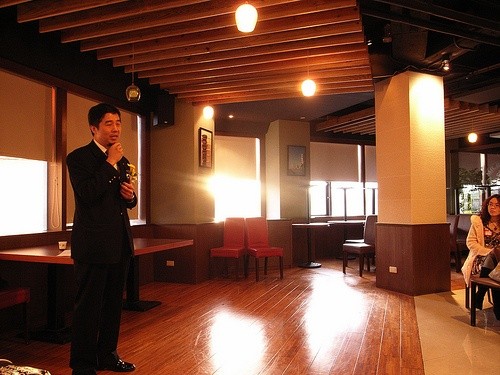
[300,42,316,98]
[203,98,213,118]
[235,1,259,33]
[441,53,450,70]
[468,112,478,143]
[126,42,141,102]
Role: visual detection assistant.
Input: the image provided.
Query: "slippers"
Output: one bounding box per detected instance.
[492,287,500,320]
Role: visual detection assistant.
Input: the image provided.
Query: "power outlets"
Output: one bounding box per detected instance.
[389,267,396,273]
[166,261,175,266]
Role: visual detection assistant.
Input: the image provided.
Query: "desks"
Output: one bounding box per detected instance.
[325,219,365,260]
[291,223,331,268]
[0,236,195,343]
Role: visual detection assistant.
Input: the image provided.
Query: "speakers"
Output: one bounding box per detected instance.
[152,94,175,127]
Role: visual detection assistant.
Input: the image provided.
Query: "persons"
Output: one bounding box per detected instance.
[461,194,500,320]
[65,102,137,375]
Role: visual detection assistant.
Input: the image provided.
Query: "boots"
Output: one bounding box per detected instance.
[474,267,492,310]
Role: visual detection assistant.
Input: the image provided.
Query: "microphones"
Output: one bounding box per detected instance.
[117,161,125,174]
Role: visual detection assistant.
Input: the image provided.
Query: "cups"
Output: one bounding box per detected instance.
[58,241,67,249]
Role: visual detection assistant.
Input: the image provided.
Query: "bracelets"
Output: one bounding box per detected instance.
[132,195,134,199]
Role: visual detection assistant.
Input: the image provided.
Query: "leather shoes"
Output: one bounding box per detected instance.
[99,360,136,371]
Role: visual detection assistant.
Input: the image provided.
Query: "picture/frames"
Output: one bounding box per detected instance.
[286,144,306,176]
[199,127,213,168]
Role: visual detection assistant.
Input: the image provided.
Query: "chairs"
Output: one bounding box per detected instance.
[445,213,500,327]
[210,218,244,282]
[342,215,378,276]
[247,217,284,281]
